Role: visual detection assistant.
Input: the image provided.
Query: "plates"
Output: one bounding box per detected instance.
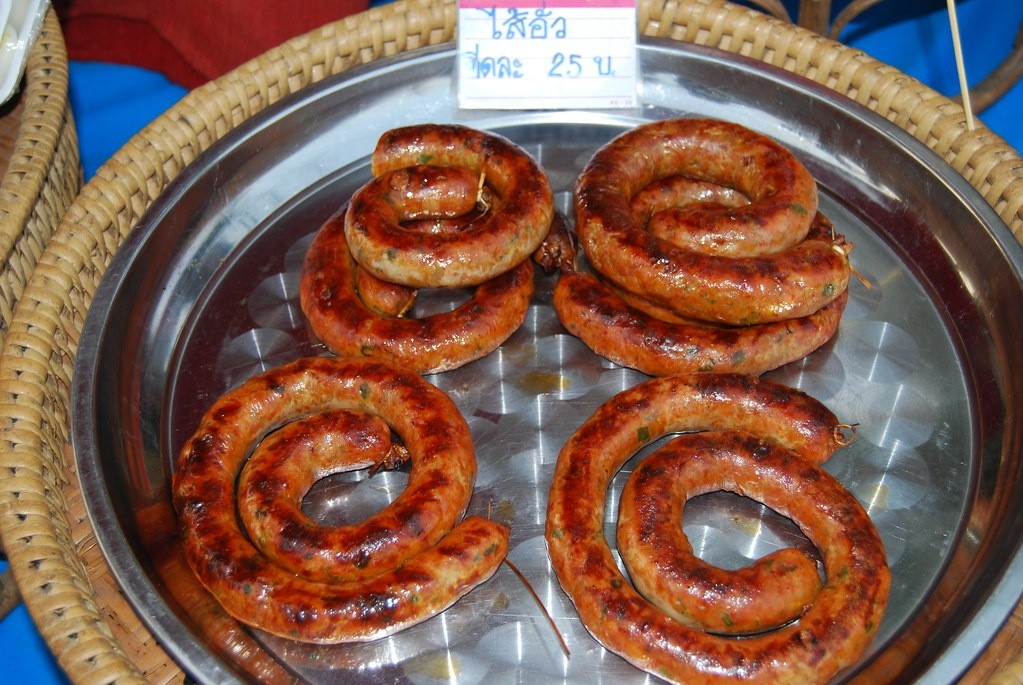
[70,35,1023,685]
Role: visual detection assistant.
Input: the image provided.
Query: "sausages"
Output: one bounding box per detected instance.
[551,116,851,379]
[544,370,891,685]
[167,355,511,645]
[300,125,580,377]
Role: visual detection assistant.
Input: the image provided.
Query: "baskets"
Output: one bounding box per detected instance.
[0,2,79,353]
[0,0,1023,685]
